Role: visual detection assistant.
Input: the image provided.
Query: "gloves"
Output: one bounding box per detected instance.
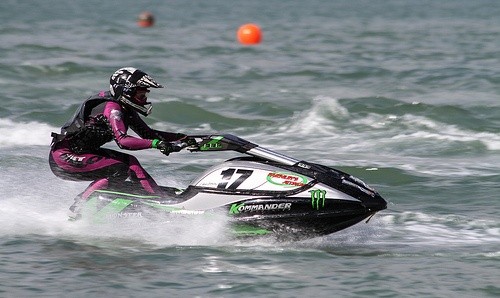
[156,140,173,156]
[185,136,197,146]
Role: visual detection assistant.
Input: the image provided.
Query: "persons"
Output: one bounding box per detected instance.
[49,67,197,226]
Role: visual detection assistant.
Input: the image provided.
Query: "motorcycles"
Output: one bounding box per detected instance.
[67,133,388,243]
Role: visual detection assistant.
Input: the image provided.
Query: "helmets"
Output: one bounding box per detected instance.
[109,67,164,117]
